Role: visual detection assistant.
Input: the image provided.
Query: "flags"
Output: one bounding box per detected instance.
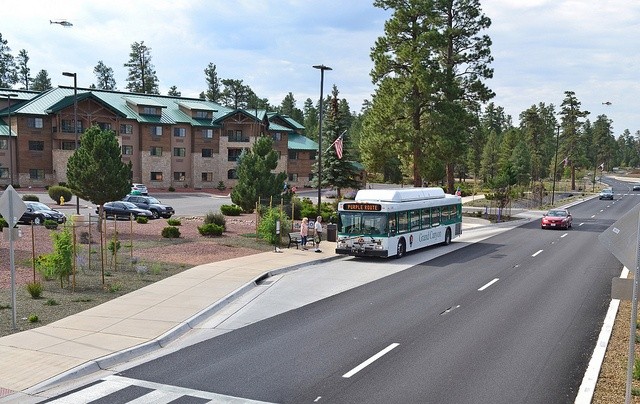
[334,134,344,160]
[563,158,569,168]
[600,164,605,171]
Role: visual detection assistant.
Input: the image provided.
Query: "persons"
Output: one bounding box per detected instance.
[314,216,323,253]
[300,217,309,250]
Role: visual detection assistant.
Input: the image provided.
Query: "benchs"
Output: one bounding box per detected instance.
[287,232,315,249]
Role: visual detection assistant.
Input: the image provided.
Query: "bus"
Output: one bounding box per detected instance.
[334,187,462,260]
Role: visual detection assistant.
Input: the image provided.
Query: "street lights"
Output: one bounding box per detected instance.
[8,94,18,186]
[312,64,333,215]
[593,144,601,192]
[552,125,562,205]
[62,72,79,214]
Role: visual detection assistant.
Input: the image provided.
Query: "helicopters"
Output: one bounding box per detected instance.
[602,102,612,105]
[50,19,73,28]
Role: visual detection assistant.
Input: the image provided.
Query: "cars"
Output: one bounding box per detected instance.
[541,208,572,230]
[599,189,614,200]
[633,185,640,191]
[19,200,66,225]
[95,201,152,220]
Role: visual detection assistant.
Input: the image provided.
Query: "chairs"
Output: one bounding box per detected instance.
[348,228,358,233]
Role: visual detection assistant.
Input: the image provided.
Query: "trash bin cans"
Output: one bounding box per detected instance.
[327,223,338,241]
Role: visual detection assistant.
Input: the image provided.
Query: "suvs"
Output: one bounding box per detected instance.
[124,196,175,219]
[131,183,148,196]
